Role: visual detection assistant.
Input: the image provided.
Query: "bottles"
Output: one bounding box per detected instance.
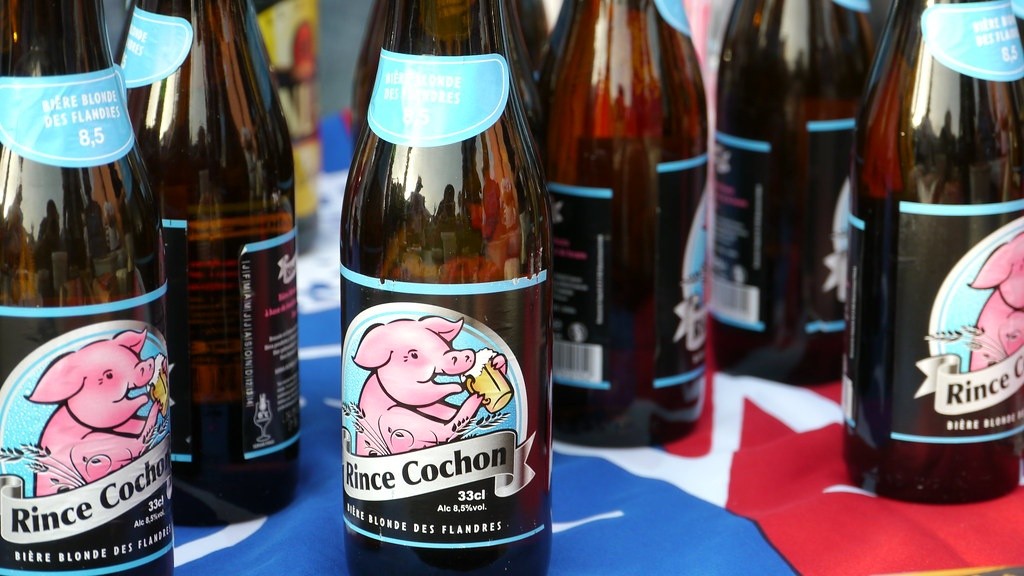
[0,0,175,576]
[352,0,547,152]
[711,0,878,388]
[341,0,551,575]
[843,0,1024,504]
[534,0,711,446]
[114,0,300,528]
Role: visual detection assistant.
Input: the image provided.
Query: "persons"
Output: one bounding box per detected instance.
[433,183,456,227]
[36,200,61,268]
